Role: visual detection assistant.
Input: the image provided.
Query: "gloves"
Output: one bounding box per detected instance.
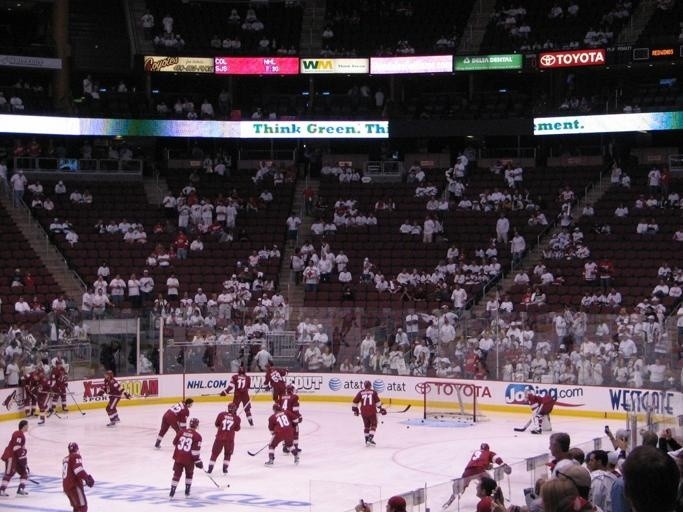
[502,464,512,474]
[380,408,387,415]
[85,474,94,487]
[124,391,131,399]
[195,460,203,467]
[96,391,104,396]
[220,389,229,396]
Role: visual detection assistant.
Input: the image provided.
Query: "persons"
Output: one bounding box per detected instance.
[63,442,95,512]
[96,370,131,426]
[2,1,682,396]
[207,403,241,474]
[22,361,68,423]
[1,420,29,495]
[356,429,682,511]
[157,399,203,496]
[264,365,302,465]
[352,381,387,446]
[221,367,254,426]
[525,391,555,434]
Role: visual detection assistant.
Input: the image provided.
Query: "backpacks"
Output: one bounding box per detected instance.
[590,471,631,512]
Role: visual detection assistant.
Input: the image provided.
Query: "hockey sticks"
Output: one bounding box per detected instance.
[97,393,130,400]
[30,479,40,484]
[359,404,411,414]
[203,469,220,488]
[247,443,269,456]
[67,387,86,415]
[514,420,533,432]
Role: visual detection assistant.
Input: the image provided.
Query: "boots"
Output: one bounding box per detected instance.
[442,498,454,507]
[0,485,9,496]
[365,434,376,445]
[155,439,161,448]
[26,408,69,425]
[249,419,253,425]
[168,486,190,497]
[106,414,120,426]
[17,483,29,495]
[265,443,301,465]
[206,469,229,473]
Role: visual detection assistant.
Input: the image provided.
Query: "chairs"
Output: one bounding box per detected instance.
[0,85,682,120]
[0,164,683,390]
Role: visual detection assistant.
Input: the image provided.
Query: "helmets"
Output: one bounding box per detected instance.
[228,403,237,411]
[273,404,281,411]
[480,443,489,451]
[286,385,294,394]
[264,363,272,370]
[239,367,245,373]
[68,442,80,453]
[364,381,372,387]
[104,371,113,379]
[37,359,62,385]
[190,418,199,430]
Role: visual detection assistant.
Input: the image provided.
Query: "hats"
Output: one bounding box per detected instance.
[670,449,683,460]
[388,496,406,510]
[647,315,655,320]
[551,459,591,488]
